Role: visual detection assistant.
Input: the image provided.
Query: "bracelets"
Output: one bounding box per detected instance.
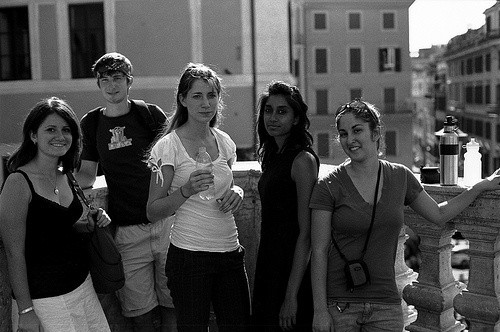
[18,305,34,316]
[180,186,191,199]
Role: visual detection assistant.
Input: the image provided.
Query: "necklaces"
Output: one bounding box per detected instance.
[33,159,60,195]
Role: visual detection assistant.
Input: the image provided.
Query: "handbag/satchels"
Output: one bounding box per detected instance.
[79,222,125,295]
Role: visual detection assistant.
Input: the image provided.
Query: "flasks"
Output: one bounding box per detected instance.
[438,115,459,186]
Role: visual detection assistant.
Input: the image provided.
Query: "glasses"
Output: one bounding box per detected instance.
[336,101,367,115]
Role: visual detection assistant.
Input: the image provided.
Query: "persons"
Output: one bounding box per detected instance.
[0,95,112,332]
[72,51,178,332]
[251,80,321,332]
[146,63,253,332]
[308,99,500,332]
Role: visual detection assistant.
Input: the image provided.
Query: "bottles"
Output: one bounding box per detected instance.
[463,138,483,187]
[196,148,216,200]
